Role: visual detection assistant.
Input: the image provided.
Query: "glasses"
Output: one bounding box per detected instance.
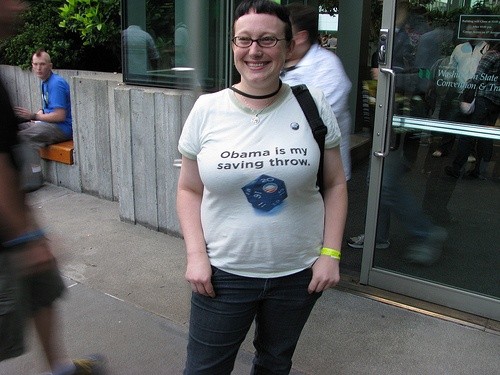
[231,36,286,48]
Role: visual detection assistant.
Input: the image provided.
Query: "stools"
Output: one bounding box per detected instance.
[38,141,74,191]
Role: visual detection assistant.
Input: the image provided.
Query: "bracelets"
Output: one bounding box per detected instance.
[6,230,44,246]
[321,248,341,259]
[32,114,36,119]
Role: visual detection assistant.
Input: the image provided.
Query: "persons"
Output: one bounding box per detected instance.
[321,34,337,47]
[123,13,159,80]
[282,3,354,182]
[175,0,347,375]
[0,0,102,375]
[348,0,500,264]
[13,52,73,192]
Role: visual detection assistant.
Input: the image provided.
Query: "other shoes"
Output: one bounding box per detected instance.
[64,353,104,375]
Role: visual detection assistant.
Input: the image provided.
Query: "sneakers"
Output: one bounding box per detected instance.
[347,233,390,248]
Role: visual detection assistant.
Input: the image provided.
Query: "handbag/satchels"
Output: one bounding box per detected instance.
[460,99,476,114]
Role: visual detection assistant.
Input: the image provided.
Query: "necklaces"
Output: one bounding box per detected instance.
[242,96,277,124]
[228,79,282,99]
